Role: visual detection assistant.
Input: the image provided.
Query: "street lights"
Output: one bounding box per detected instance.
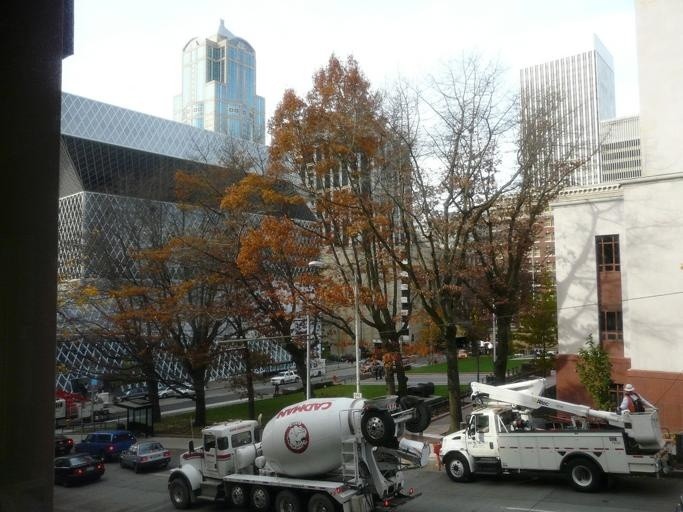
[308,261,360,393]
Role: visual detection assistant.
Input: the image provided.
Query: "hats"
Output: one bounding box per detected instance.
[623,384,636,391]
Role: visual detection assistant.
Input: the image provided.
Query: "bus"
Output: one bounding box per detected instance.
[293,360,327,377]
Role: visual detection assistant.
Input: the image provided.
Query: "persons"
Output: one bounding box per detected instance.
[433,441,445,471]
[616,384,660,413]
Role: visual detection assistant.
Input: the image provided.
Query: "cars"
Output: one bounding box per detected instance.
[54,430,172,487]
[270,371,300,385]
[112,385,206,403]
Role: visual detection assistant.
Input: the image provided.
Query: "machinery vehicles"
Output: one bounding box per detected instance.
[56,391,104,427]
[439,378,677,492]
[168,396,430,512]
